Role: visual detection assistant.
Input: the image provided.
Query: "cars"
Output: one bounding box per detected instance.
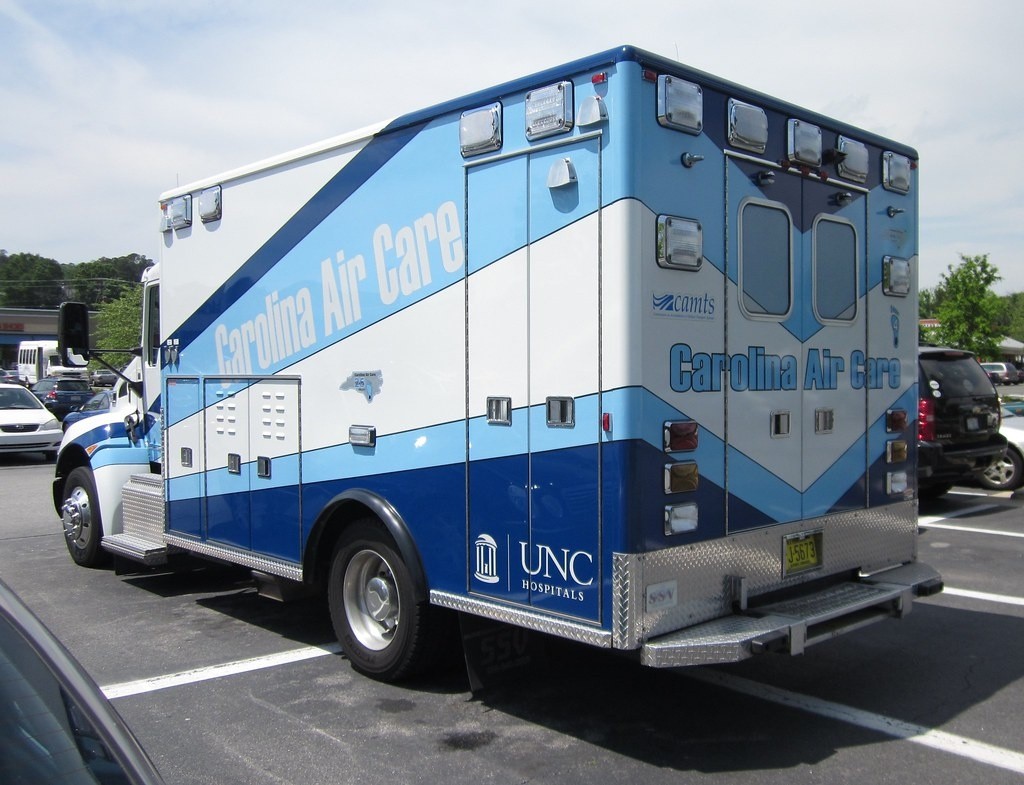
[988,372,1001,385]
[0,368,20,384]
[1,579,166,785]
[974,402,1024,491]
[30,376,96,419]
[0,384,64,463]
[62,389,117,433]
[90,370,117,387]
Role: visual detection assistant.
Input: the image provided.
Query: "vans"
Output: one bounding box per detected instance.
[980,363,1019,386]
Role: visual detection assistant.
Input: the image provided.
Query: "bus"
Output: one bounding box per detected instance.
[18,340,90,389]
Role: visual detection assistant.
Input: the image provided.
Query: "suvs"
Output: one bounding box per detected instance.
[918,341,1009,500]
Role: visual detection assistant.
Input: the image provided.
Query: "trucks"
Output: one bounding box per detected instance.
[50,49,944,697]
